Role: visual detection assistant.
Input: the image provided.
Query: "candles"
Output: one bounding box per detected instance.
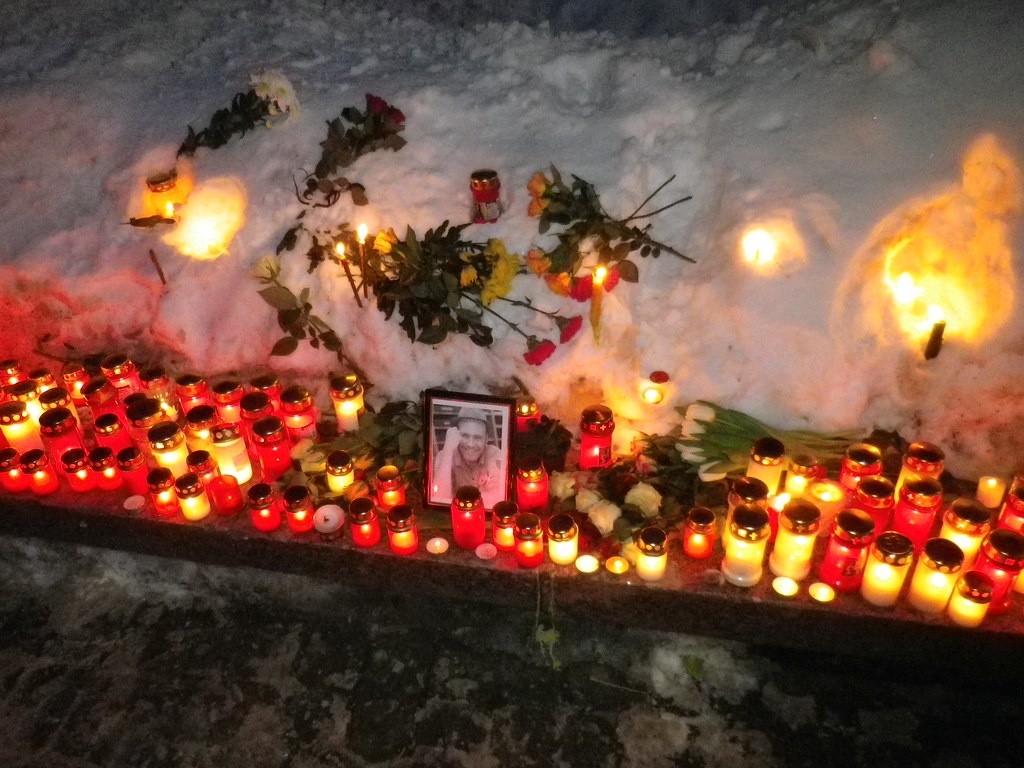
[976,476,1006,508]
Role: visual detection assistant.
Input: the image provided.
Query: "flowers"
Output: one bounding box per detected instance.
[177,67,300,160]
[250,91,697,387]
[548,399,981,567]
[275,401,574,507]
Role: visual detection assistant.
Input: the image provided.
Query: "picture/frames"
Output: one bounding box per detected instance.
[422,389,516,516]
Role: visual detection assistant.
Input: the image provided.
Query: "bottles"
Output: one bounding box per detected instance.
[0,351,1024,637]
[470,170,500,224]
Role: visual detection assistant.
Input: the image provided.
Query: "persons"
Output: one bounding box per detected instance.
[432,407,502,502]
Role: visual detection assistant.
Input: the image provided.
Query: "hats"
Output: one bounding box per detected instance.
[451,408,494,433]
